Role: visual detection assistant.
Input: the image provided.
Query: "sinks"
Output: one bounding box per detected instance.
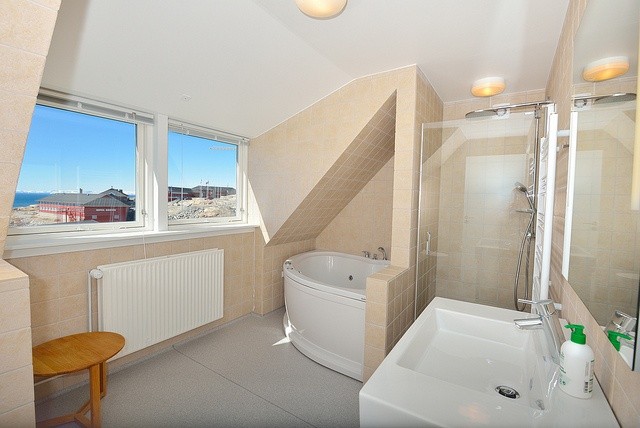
[396,308,553,412]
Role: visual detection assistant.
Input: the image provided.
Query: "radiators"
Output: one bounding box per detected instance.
[98,248,225,364]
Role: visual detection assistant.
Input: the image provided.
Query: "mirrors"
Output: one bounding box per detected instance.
[560,1,640,371]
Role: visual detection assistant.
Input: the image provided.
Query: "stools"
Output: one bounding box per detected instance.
[31,332,126,427]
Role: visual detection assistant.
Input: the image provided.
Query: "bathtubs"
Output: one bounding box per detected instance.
[282,250,391,383]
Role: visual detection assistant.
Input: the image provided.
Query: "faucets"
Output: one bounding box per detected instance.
[378,246,388,261]
[513,299,566,365]
[604,308,637,350]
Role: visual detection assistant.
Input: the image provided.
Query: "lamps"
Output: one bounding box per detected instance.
[471,77,507,97]
[582,54,630,83]
[297,0,347,20]
